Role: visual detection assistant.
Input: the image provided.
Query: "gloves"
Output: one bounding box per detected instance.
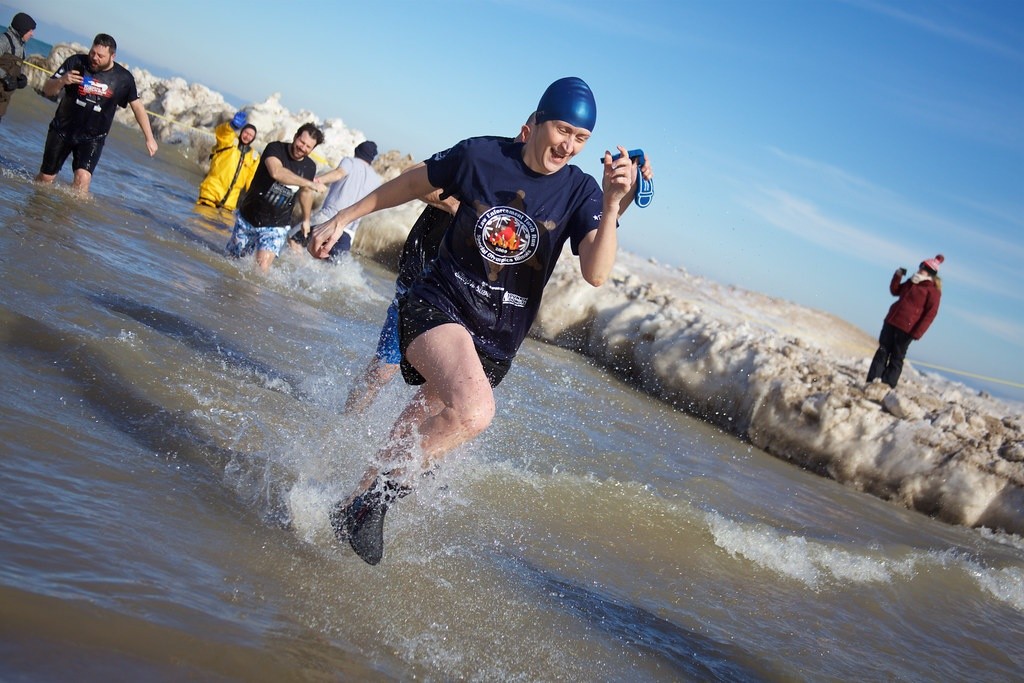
[1,77,15,91]
[18,75,28,90]
[231,111,247,131]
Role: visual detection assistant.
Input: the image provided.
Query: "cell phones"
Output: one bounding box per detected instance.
[73,63,85,79]
[900,268,906,276]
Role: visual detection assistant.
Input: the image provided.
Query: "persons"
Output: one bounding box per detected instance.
[285,140,385,261]
[304,75,633,564]
[224,122,328,268]
[866,254,944,389]
[0,12,35,122]
[195,109,261,210]
[340,110,653,413]
[34,33,158,193]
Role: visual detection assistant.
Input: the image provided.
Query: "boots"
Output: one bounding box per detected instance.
[345,473,407,569]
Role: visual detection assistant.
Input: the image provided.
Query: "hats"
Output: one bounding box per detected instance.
[355,140,377,164]
[920,256,944,276]
[11,12,36,38]
